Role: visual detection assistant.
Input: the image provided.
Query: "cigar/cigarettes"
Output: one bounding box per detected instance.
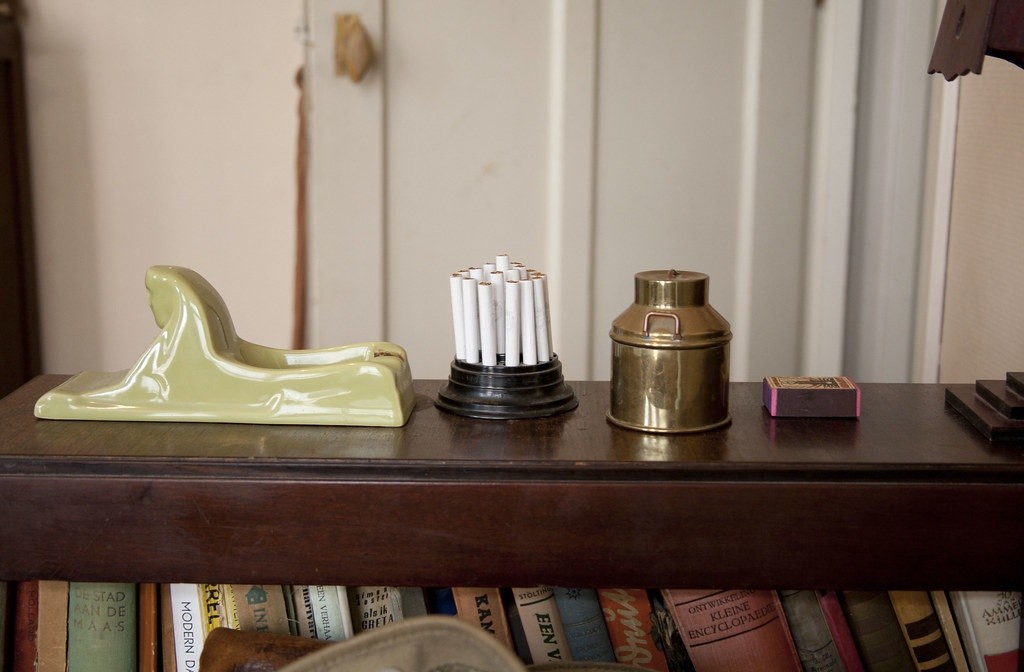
[447,254,551,371]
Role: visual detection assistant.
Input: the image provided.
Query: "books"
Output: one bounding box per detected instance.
[1,580,1023,671]
